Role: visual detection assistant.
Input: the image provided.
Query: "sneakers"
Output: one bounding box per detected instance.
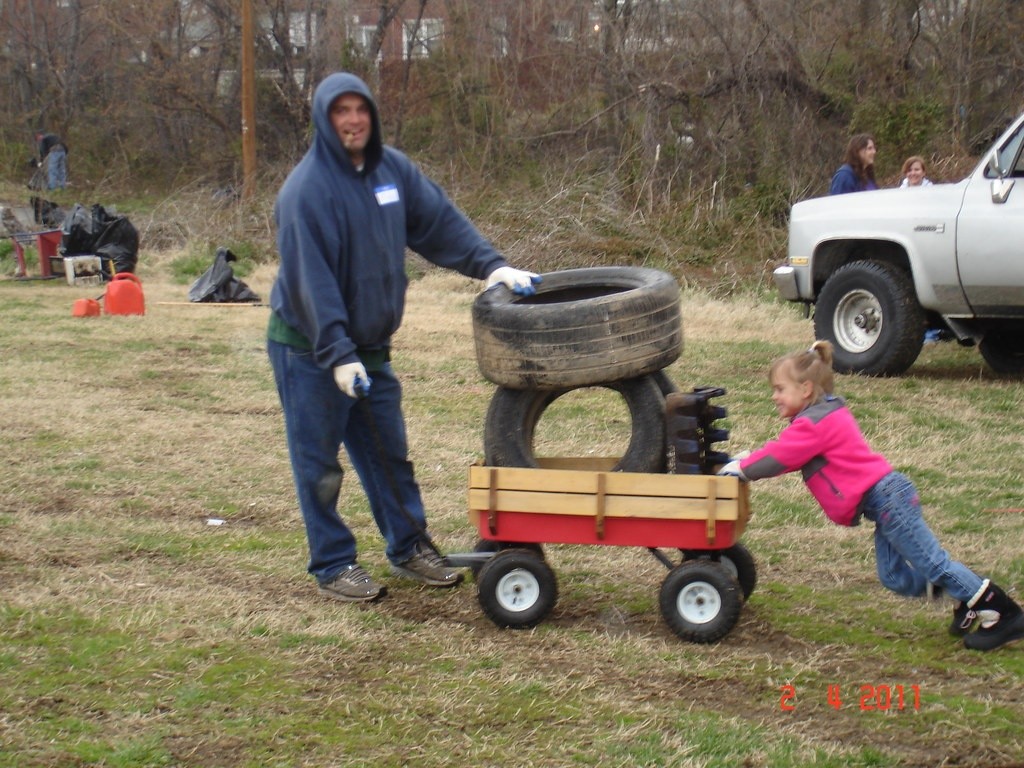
[317,562,388,603]
[390,543,466,587]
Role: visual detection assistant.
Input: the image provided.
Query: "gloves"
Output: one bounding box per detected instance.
[716,460,750,482]
[333,362,372,400]
[732,449,752,460]
[36,163,42,167]
[484,266,541,296]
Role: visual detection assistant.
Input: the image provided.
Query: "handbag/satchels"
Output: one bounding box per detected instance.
[27,167,46,191]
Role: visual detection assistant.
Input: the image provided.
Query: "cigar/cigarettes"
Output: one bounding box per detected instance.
[344,133,353,147]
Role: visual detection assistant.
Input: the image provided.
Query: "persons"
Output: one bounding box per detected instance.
[35,130,69,191]
[830,134,879,196]
[901,156,942,343]
[719,341,1024,652]
[268,74,543,601]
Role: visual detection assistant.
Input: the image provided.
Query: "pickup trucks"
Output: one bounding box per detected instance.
[772,113,1024,378]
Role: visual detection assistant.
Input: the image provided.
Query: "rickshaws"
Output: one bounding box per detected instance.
[438,463,757,643]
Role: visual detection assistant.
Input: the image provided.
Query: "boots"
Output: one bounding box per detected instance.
[948,599,977,638]
[963,579,1024,652]
